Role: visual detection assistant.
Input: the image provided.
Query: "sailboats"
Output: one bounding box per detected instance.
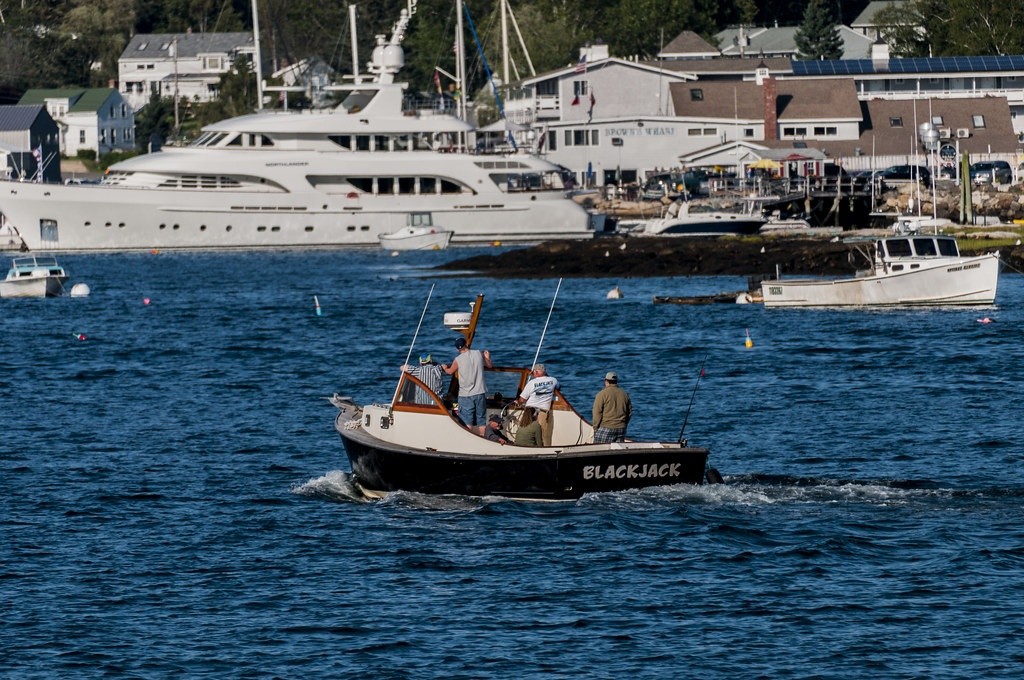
[869,97,1023,229]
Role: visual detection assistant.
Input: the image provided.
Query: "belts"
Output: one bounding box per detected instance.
[536,408,550,415]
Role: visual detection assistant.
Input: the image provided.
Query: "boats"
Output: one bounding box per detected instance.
[760,235,1000,308]
[0,0,606,253]
[617,200,766,236]
[653,272,780,304]
[0,254,69,298]
[319,277,711,500]
[378,226,455,250]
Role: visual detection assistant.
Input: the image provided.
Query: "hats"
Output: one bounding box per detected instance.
[531,364,545,375]
[454,338,466,349]
[601,372,617,380]
[419,352,432,366]
[488,414,505,423]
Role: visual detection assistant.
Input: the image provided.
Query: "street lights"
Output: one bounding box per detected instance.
[168,39,180,129]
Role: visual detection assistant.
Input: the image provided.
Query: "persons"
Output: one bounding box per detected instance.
[400,354,447,406]
[441,337,515,446]
[513,364,562,446]
[592,372,632,444]
[516,406,542,447]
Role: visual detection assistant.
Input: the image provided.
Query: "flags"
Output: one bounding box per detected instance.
[434,73,444,95]
[576,55,586,73]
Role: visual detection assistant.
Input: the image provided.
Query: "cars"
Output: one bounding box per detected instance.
[632,161,1012,197]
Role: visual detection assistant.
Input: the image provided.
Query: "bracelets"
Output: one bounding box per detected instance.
[444,367,448,371]
